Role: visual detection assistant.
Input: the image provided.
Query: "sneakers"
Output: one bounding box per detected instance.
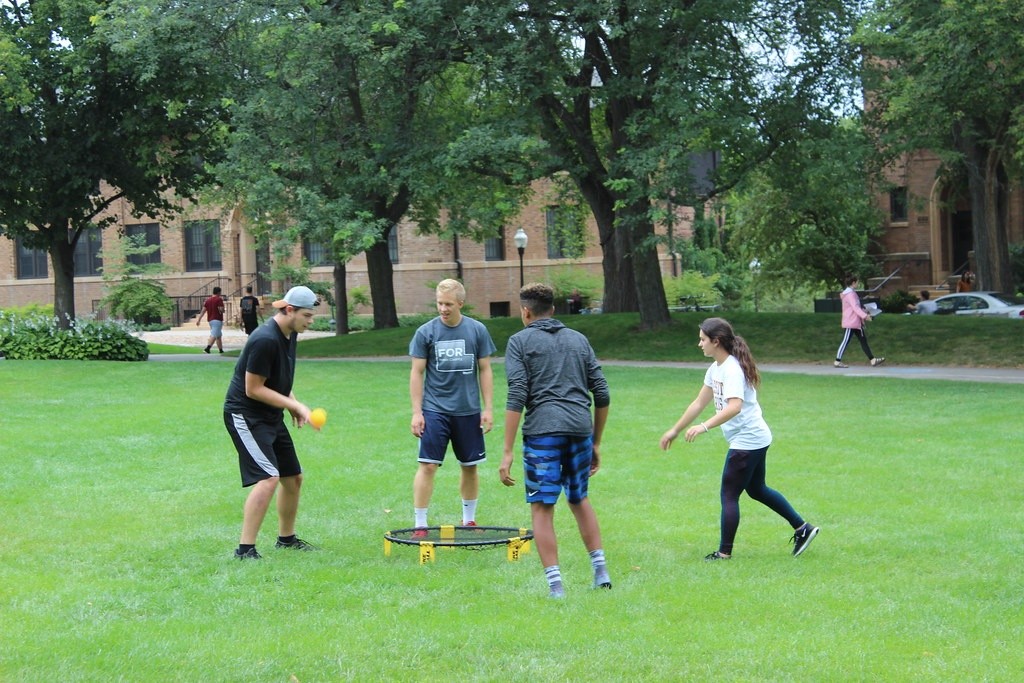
[790,524,820,557]
[706,553,728,561]
[276,538,313,553]
[234,548,262,559]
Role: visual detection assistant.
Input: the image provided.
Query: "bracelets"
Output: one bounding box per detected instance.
[701,422,708,432]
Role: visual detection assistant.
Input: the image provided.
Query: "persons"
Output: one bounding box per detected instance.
[238,285,262,337]
[956,272,970,292]
[658,317,820,563]
[498,283,612,601]
[196,287,227,354]
[222,284,321,561]
[408,278,498,540]
[833,274,886,368]
[907,291,939,316]
[565,288,582,315]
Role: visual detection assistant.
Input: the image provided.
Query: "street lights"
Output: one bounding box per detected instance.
[513,226,528,288]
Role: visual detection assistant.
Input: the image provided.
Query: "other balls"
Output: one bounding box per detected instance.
[310,408,327,428]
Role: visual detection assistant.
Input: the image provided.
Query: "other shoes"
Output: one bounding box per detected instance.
[834,361,849,368]
[410,530,429,540]
[203,349,211,354]
[870,357,885,366]
[467,520,479,533]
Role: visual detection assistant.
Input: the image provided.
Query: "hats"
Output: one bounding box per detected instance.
[273,286,315,311]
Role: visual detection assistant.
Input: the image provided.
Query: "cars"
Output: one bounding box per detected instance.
[903,290,1024,322]
